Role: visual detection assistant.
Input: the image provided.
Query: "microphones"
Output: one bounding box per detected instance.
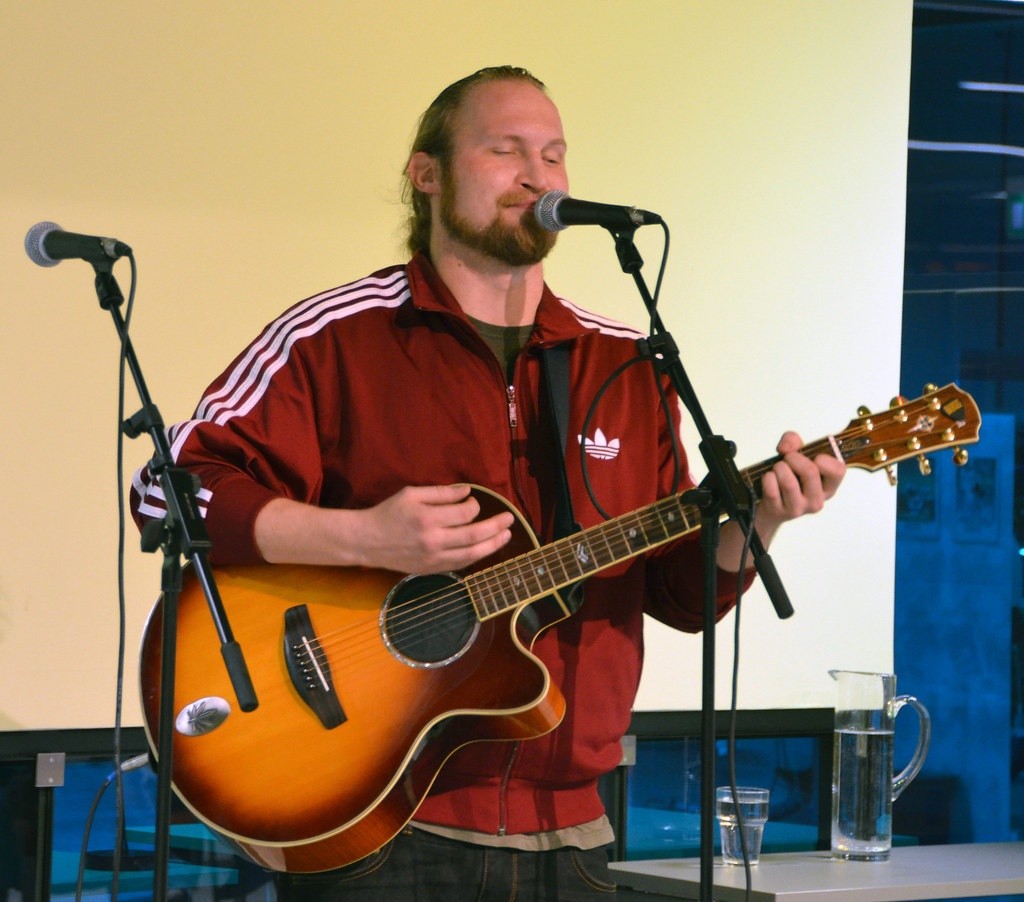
[24,222,132,268]
[534,189,662,230]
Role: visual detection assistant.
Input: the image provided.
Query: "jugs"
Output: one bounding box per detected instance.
[828,668,930,863]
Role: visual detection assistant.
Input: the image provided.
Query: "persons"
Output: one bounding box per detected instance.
[128,66,848,902]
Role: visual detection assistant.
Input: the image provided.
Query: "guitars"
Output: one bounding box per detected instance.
[135,380,984,875]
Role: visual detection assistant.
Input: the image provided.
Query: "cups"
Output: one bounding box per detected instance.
[716,786,769,865]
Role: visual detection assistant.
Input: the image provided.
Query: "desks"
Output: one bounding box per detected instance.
[607,842,1024,902]
